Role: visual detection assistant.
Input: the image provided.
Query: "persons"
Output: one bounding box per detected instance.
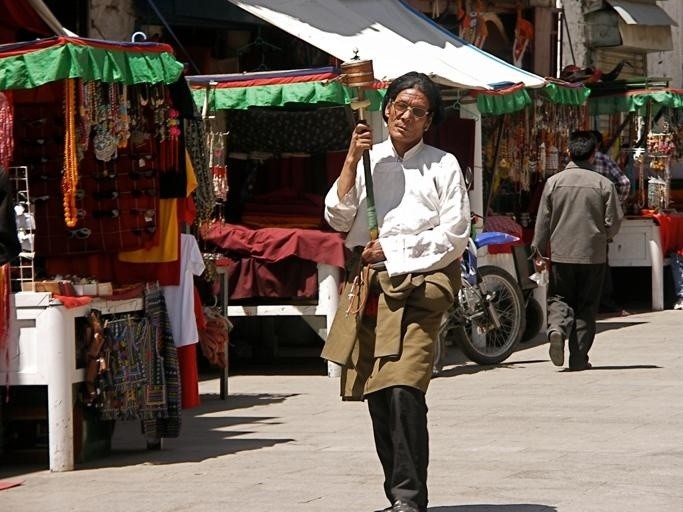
[594,130,631,203]
[531,133,623,370]
[325,72,472,511]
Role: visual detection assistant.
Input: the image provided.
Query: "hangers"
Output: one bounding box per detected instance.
[441,88,481,122]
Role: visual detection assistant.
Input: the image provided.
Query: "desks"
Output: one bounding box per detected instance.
[592,214,683,312]
[2,293,145,472]
[192,265,231,403]
[226,232,348,379]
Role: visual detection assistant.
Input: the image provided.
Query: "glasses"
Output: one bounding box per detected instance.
[392,101,429,118]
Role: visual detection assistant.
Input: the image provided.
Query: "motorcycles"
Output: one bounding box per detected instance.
[425,165,527,377]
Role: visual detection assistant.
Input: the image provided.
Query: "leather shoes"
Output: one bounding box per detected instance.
[374,505,427,512]
[549,330,564,366]
[569,362,592,371]
[394,498,420,512]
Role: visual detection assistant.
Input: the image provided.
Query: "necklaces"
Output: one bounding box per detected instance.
[94,318,168,444]
[0,102,13,170]
[62,74,233,227]
